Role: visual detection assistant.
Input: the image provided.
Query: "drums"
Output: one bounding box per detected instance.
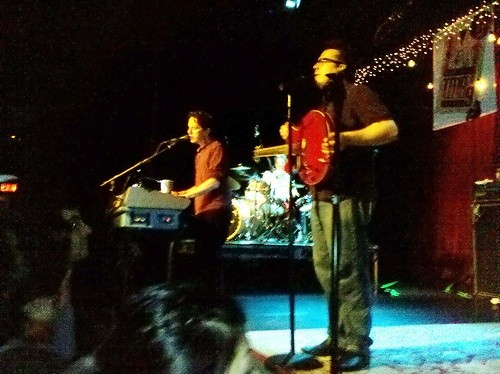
[246,180,271,198]
[228,200,249,241]
[294,196,311,212]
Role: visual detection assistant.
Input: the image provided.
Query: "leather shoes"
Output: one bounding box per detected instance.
[337,353,370,371]
[301,337,333,357]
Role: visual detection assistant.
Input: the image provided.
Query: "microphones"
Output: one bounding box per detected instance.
[162,135,189,144]
[279,76,305,90]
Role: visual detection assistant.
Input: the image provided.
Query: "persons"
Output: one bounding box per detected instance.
[279,39,398,372]
[0,201,262,374]
[169,109,232,311]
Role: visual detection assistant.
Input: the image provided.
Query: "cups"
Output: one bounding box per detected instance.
[160,179,174,194]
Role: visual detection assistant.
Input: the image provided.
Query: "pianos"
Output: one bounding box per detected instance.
[111,186,191,231]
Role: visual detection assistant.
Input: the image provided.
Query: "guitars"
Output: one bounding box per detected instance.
[253,107,337,186]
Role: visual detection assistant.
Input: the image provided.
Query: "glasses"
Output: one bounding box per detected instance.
[65,213,84,232]
[315,58,339,64]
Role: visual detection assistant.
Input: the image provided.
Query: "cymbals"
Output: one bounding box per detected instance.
[229,167,251,170]
